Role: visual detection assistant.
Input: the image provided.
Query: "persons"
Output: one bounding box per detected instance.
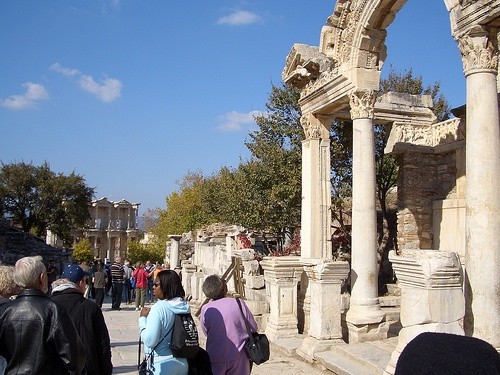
[199,274,258,375]
[109,256,171,311]
[394,332,500,375]
[0,252,114,375]
[138,270,191,375]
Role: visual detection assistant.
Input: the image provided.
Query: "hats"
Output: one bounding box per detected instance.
[60,264,91,283]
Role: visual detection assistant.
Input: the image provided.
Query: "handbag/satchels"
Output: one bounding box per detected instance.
[243,332,270,365]
[131,277,136,288]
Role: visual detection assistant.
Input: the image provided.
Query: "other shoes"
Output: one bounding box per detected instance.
[112,307,122,310]
[136,308,140,310]
[141,307,144,310]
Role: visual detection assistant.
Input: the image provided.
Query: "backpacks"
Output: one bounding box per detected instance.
[105,264,111,274]
[168,314,200,358]
[144,265,153,280]
